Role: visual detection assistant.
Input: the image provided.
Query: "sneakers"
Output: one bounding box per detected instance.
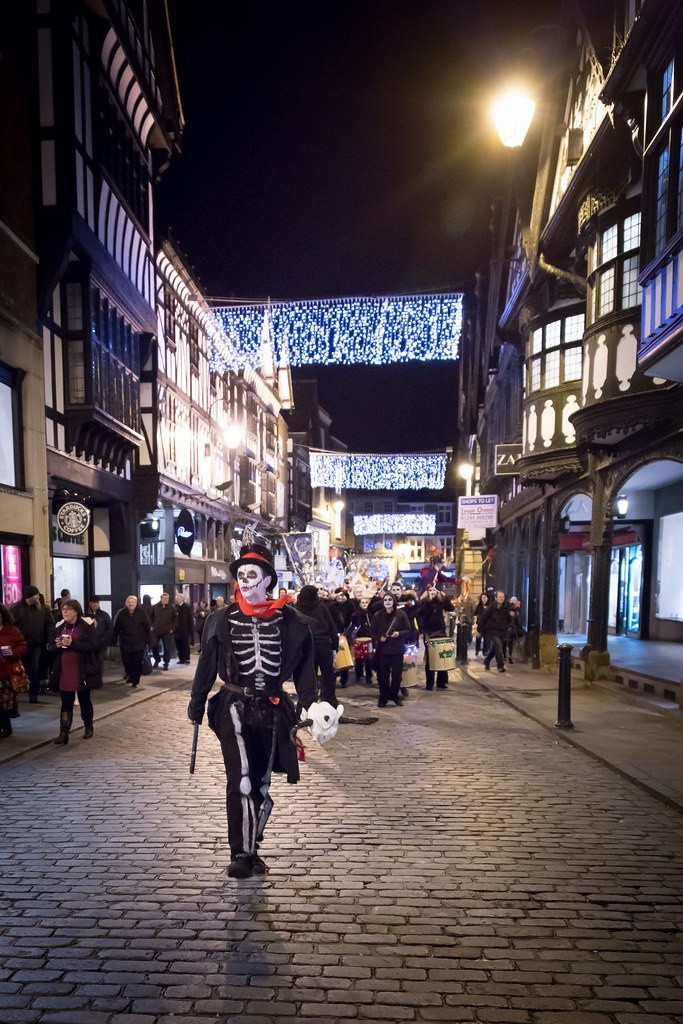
[252,857,266,875]
[227,861,253,879]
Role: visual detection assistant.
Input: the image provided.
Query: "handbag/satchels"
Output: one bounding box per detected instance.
[141,646,153,675]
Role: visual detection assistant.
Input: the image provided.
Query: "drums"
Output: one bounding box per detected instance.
[353,637,374,661]
[426,637,457,671]
[398,643,418,688]
[332,634,354,671]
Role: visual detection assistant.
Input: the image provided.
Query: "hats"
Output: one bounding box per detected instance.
[23,585,39,600]
[229,544,278,592]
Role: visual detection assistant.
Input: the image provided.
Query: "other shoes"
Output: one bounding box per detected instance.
[400,687,409,696]
[497,667,505,674]
[378,699,388,708]
[394,696,404,706]
[367,678,373,684]
[357,675,364,680]
[427,685,434,690]
[340,679,348,687]
[484,661,491,672]
[436,683,448,690]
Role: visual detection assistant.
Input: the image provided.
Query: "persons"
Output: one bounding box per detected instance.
[416,586,455,691]
[293,586,339,710]
[83,595,113,689]
[0,586,100,745]
[278,582,457,609]
[188,543,319,879]
[113,592,235,689]
[328,588,361,689]
[371,593,411,707]
[341,596,374,687]
[460,589,521,673]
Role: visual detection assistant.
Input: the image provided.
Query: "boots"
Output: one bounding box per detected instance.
[53,711,73,744]
[81,705,94,739]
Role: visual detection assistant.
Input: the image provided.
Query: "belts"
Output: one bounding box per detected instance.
[227,683,283,696]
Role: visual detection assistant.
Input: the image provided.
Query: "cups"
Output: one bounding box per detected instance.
[1,646,8,656]
[61,635,69,648]
